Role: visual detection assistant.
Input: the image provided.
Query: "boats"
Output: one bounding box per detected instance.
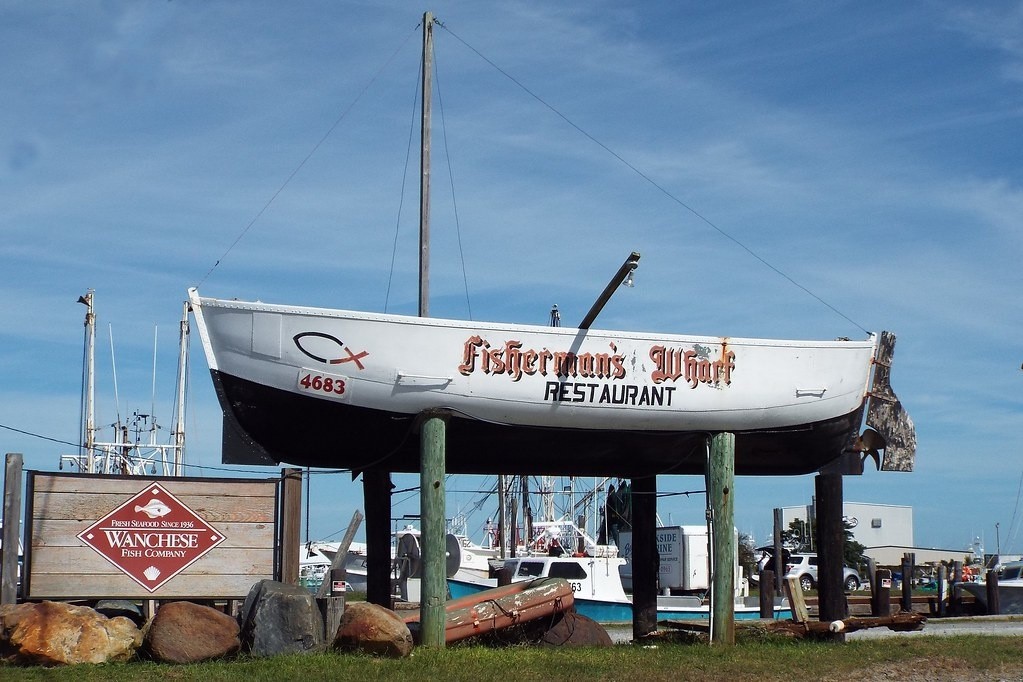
[386,524,796,645]
[0,518,25,601]
[299,543,333,599]
[954,476,1022,615]
[314,540,403,599]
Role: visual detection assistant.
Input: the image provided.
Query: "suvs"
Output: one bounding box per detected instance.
[782,553,863,594]
